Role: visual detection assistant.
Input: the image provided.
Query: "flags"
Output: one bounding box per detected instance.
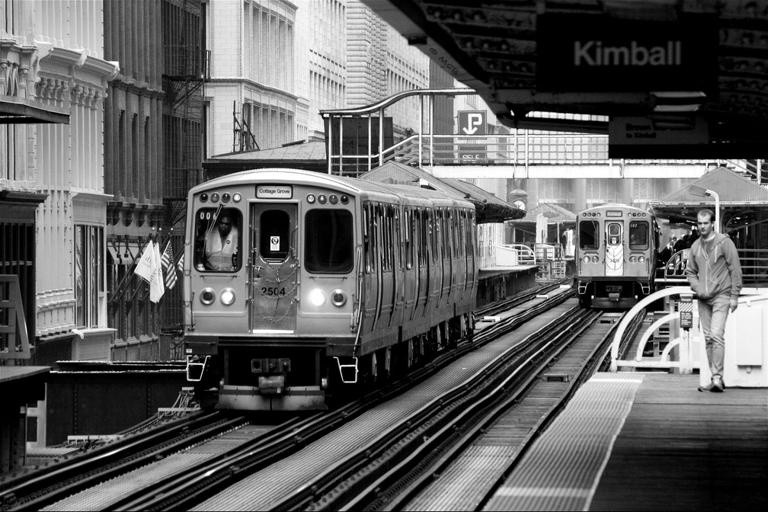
[176,251,185,273]
[149,240,165,303]
[133,239,164,304]
[160,240,178,291]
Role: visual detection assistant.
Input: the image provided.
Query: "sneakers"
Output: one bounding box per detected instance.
[698,376,727,393]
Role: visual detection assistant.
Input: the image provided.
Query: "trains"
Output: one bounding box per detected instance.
[184,169,479,412]
[576,204,660,309]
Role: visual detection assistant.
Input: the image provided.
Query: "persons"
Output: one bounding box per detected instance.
[659,229,699,266]
[686,208,743,393]
[202,210,237,272]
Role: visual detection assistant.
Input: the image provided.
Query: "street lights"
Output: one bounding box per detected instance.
[705,189,721,233]
